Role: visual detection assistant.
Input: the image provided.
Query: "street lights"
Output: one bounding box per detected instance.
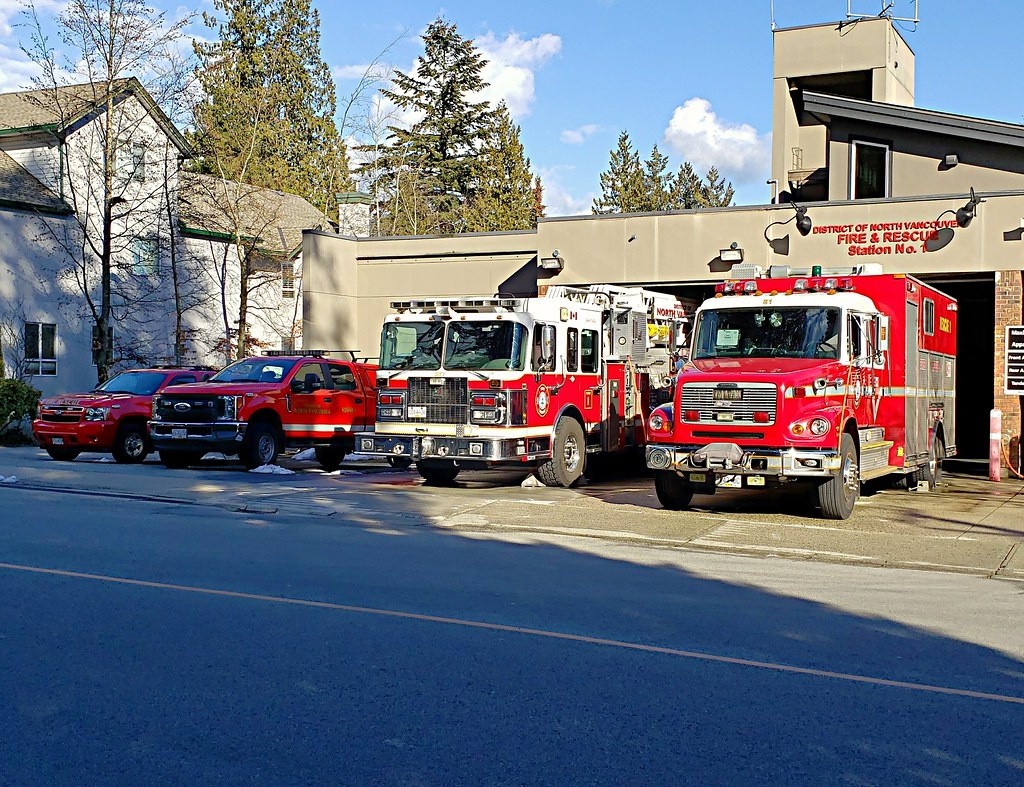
[766,180,781,205]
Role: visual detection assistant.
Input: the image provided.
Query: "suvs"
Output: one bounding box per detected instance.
[31,364,239,466]
[147,348,414,472]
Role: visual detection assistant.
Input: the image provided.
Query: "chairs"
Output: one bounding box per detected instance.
[261,371,276,380]
[304,373,320,383]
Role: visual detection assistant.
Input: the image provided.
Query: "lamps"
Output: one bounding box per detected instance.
[946,154,957,165]
[790,201,812,236]
[720,242,744,263]
[955,187,981,228]
[541,250,564,270]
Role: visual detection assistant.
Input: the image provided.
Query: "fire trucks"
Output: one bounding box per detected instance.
[644,262,959,522]
[349,284,704,488]
